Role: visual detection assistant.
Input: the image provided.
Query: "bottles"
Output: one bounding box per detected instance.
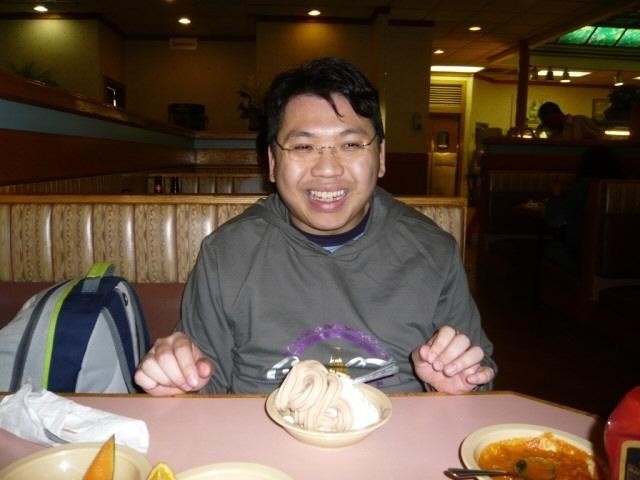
[153,175,181,193]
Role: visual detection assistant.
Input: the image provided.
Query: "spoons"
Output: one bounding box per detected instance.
[447,456,557,480]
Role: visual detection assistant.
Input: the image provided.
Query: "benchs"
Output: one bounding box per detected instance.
[484,190,579,257]
[598,211,640,319]
[0,281,188,353]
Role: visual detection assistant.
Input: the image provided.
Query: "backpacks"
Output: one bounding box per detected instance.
[0,262,149,395]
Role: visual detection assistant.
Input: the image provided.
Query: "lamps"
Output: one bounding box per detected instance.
[613,74,625,87]
[529,68,538,80]
[560,69,571,82]
[544,68,554,81]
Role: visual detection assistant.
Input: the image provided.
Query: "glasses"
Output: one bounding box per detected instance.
[275,133,377,162]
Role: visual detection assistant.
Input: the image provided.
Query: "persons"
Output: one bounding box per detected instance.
[538,101,604,137]
[133,55,500,398]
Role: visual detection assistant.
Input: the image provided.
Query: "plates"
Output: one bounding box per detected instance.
[0,440,164,480]
[264,382,394,448]
[164,461,296,480]
[458,422,622,479]
